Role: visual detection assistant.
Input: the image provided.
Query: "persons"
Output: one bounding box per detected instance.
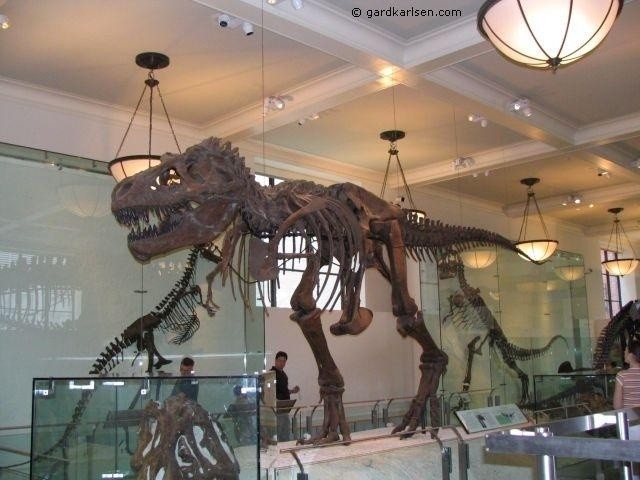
[171,356,199,401]
[270,350,300,400]
[614,341,640,480]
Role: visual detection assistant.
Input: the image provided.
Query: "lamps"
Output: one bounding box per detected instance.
[477,0,624,75]
[219,14,254,36]
[380,130,426,223]
[513,97,533,117]
[461,249,499,269]
[260,95,285,118]
[553,265,585,281]
[561,158,640,208]
[600,208,640,277]
[467,114,488,128]
[514,178,559,262]
[107,51,182,183]
[449,156,474,172]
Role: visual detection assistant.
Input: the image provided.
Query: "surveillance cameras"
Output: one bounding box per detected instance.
[395,196,406,202]
